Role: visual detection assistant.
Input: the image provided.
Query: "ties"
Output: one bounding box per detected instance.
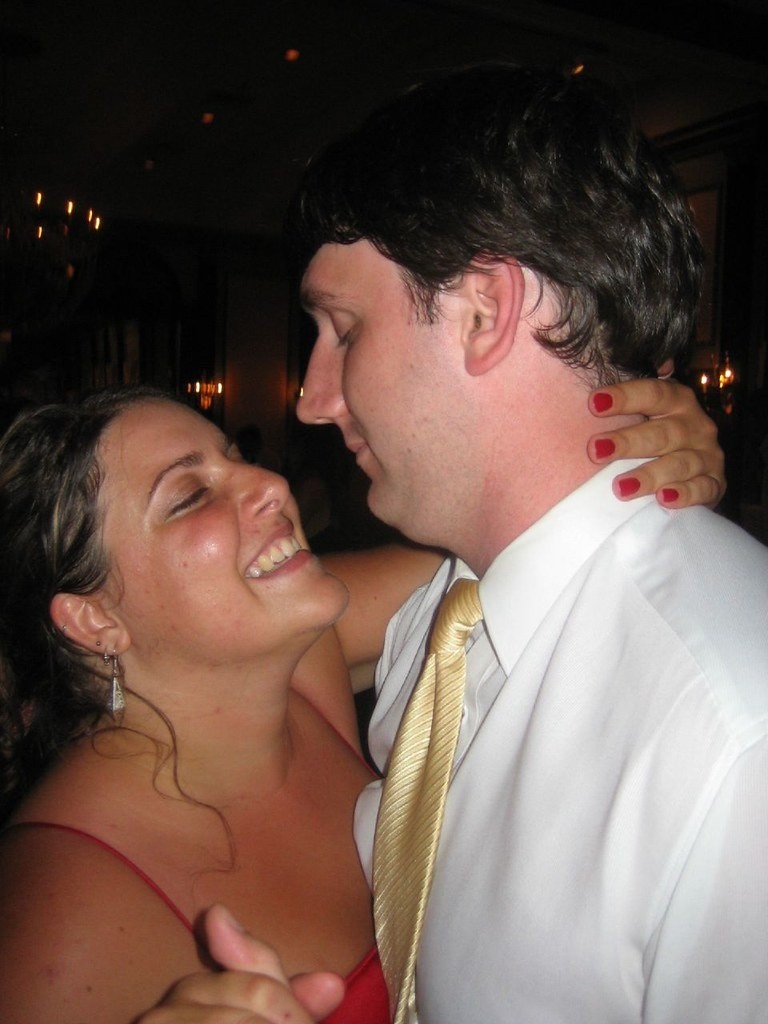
[374,576,482,1024]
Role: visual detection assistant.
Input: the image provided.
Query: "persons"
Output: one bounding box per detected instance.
[135,54,768,1024]
[0,354,726,1024]
